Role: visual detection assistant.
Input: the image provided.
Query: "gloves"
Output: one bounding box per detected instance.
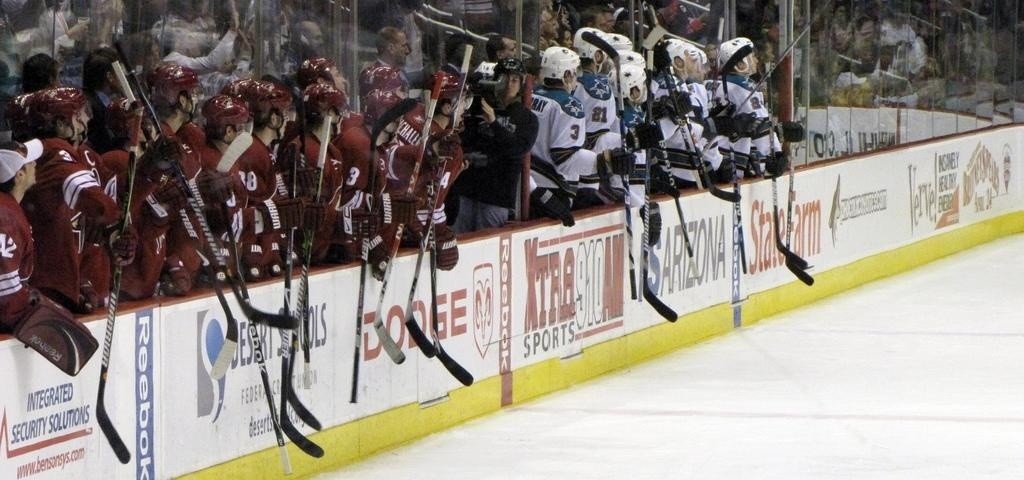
[252,164,328,234]
[159,254,194,297]
[531,187,576,227]
[138,132,245,220]
[640,200,661,245]
[113,223,139,268]
[597,147,637,181]
[80,183,121,228]
[659,91,694,125]
[765,151,790,177]
[626,123,664,151]
[717,159,739,184]
[706,113,803,143]
[335,191,459,280]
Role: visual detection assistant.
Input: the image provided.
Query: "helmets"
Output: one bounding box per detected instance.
[493,58,526,84]
[424,71,469,105]
[104,97,147,140]
[5,87,86,131]
[143,57,405,126]
[665,37,755,78]
[538,27,649,104]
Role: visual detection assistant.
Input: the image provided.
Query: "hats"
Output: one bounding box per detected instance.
[1,135,43,185]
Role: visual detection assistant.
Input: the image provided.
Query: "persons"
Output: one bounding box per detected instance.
[0,0,806,376]
[809,1,1024,125]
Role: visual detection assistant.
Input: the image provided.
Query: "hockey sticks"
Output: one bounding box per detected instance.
[425,142,475,386]
[401,43,473,359]
[351,98,419,405]
[765,59,809,270]
[95,102,145,467]
[582,23,814,324]
[96,44,473,474]
[623,1,742,260]
[723,44,754,276]
[111,59,240,381]
[784,78,814,287]
[127,67,334,474]
[582,30,638,301]
[640,48,678,323]
